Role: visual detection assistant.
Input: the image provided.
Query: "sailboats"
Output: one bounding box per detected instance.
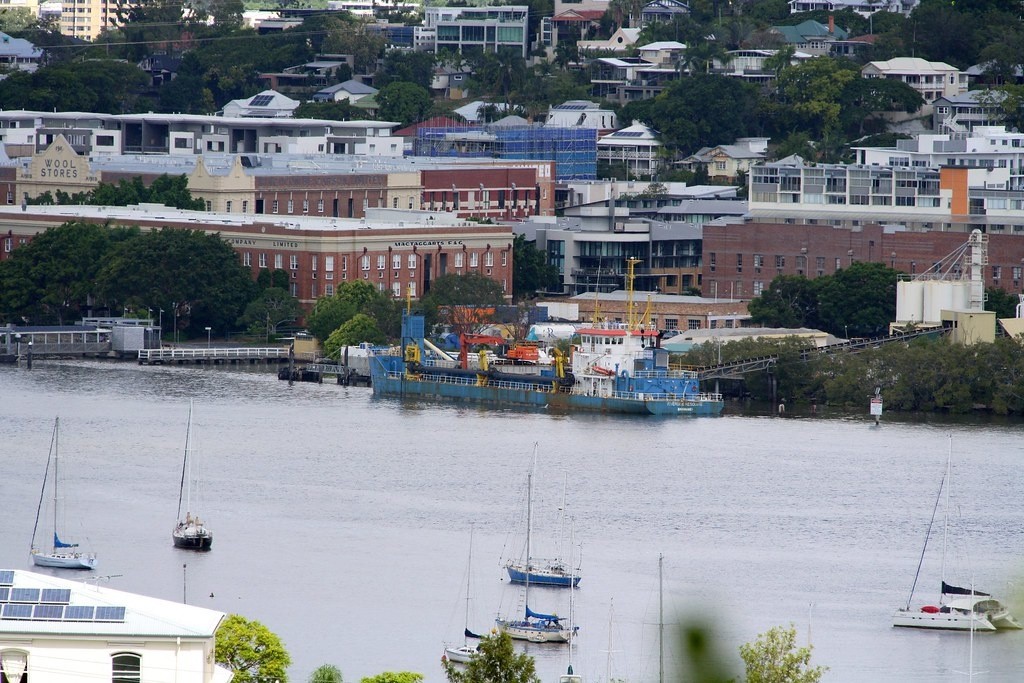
[172,394,213,550]
[495,439,582,587]
[28,416,97,571]
[446,521,487,664]
[493,473,581,643]
[891,431,1022,632]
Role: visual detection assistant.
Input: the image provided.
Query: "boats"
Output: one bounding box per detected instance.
[365,256,725,419]
[0,353,19,363]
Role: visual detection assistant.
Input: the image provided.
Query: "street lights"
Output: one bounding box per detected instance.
[148,307,154,320]
[205,326,211,349]
[159,307,165,326]
[174,309,181,345]
